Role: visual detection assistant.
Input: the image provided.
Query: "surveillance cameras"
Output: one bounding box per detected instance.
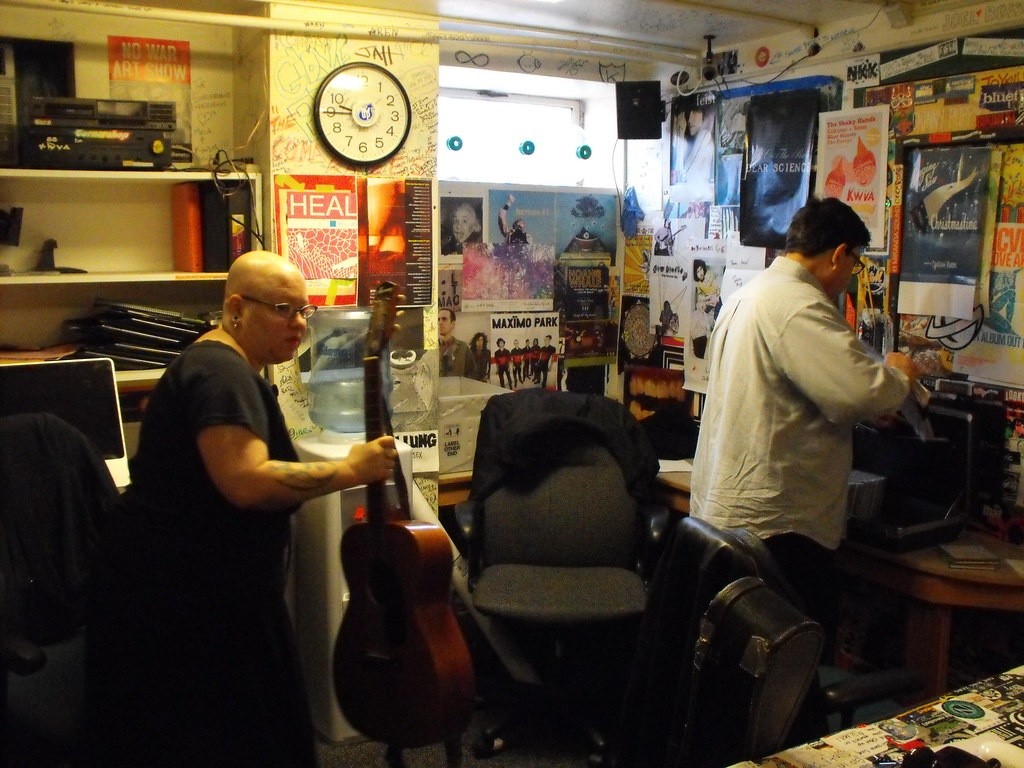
[701,57,718,80]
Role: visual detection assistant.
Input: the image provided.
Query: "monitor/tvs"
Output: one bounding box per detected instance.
[0,358,133,487]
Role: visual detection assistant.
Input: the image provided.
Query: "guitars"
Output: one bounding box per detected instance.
[332,277,477,748]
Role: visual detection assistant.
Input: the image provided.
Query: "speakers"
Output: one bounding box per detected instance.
[616,80,663,139]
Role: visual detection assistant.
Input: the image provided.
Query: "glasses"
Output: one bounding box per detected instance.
[242,295,317,319]
[833,241,865,275]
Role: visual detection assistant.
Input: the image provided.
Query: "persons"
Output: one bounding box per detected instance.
[686,196,918,737]
[693,257,722,318]
[495,335,556,390]
[689,293,712,360]
[469,331,490,383]
[498,193,532,244]
[442,202,483,255]
[680,97,713,184]
[120,248,398,767]
[436,308,476,379]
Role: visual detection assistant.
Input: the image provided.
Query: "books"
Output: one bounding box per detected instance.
[73,301,213,371]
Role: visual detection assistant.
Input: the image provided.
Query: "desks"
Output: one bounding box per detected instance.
[436,468,476,535]
[653,455,1024,698]
[725,664,1024,768]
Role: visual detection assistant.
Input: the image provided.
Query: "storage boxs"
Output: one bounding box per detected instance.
[439,373,512,472]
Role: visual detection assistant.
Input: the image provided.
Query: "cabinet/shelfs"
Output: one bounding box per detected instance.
[0,160,263,428]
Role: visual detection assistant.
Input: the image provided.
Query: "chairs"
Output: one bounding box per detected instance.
[0,413,184,768]
[665,577,824,768]
[457,392,674,768]
[659,518,931,748]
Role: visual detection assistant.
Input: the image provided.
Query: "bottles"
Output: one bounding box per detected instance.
[305,305,394,435]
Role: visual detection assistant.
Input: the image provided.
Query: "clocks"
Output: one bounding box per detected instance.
[314,62,412,165]
